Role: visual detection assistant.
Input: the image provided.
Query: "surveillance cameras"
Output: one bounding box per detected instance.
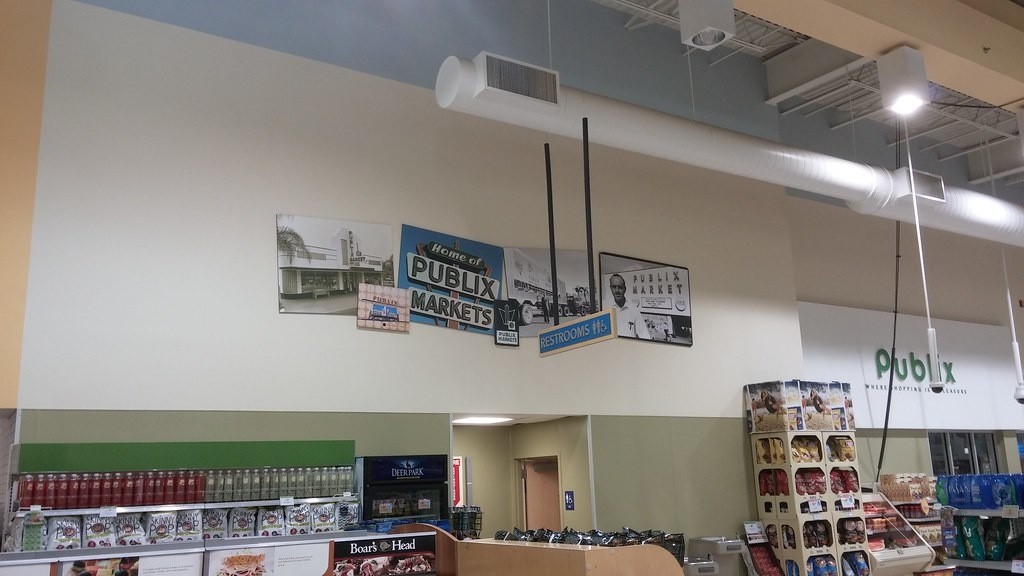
[1016,398,1024,404]
[932,387,943,393]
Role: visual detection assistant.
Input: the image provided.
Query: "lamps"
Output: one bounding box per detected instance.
[876,46,931,115]
[679,0,736,52]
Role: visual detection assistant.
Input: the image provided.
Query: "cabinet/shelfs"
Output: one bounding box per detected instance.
[944,509,1024,576]
[861,482,956,576]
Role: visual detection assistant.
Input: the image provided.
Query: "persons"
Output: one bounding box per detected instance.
[751,398,757,417]
[663,322,671,341]
[603,274,651,339]
[541,291,552,324]
[845,397,850,413]
[802,394,807,413]
[810,389,829,413]
[761,391,783,414]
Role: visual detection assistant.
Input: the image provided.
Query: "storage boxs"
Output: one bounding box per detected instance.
[773,431,831,520]
[778,513,839,576]
[822,431,863,515]
[879,473,937,501]
[831,509,872,576]
[759,519,784,573]
[913,523,942,544]
[750,434,776,520]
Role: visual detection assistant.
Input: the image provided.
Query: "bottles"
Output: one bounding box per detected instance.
[18,471,205,509]
[206,465,354,502]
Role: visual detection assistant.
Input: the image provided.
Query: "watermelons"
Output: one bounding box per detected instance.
[868,537,917,552]
